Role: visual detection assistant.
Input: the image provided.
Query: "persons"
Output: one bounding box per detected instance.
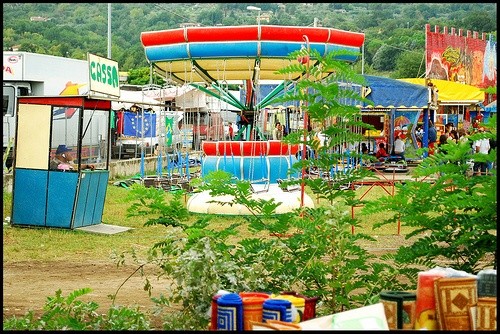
[376,143,390,159]
[226,122,233,140]
[55,145,95,171]
[362,143,368,153]
[389,136,405,161]
[273,122,285,140]
[300,125,330,151]
[416,123,496,175]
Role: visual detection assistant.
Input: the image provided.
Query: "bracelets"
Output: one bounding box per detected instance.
[87,165,89,169]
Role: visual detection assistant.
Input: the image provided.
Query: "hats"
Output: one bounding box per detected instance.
[56,145,72,155]
[440,135,447,143]
[363,148,368,153]
